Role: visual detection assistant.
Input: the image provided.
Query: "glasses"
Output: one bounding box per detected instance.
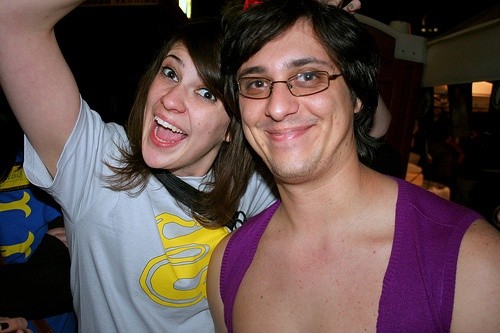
[236,70,347,100]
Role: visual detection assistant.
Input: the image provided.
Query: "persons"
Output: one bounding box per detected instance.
[405,102,491,210]
[0,0,391,333]
[207,0,500,332]
[0,96,130,333]
